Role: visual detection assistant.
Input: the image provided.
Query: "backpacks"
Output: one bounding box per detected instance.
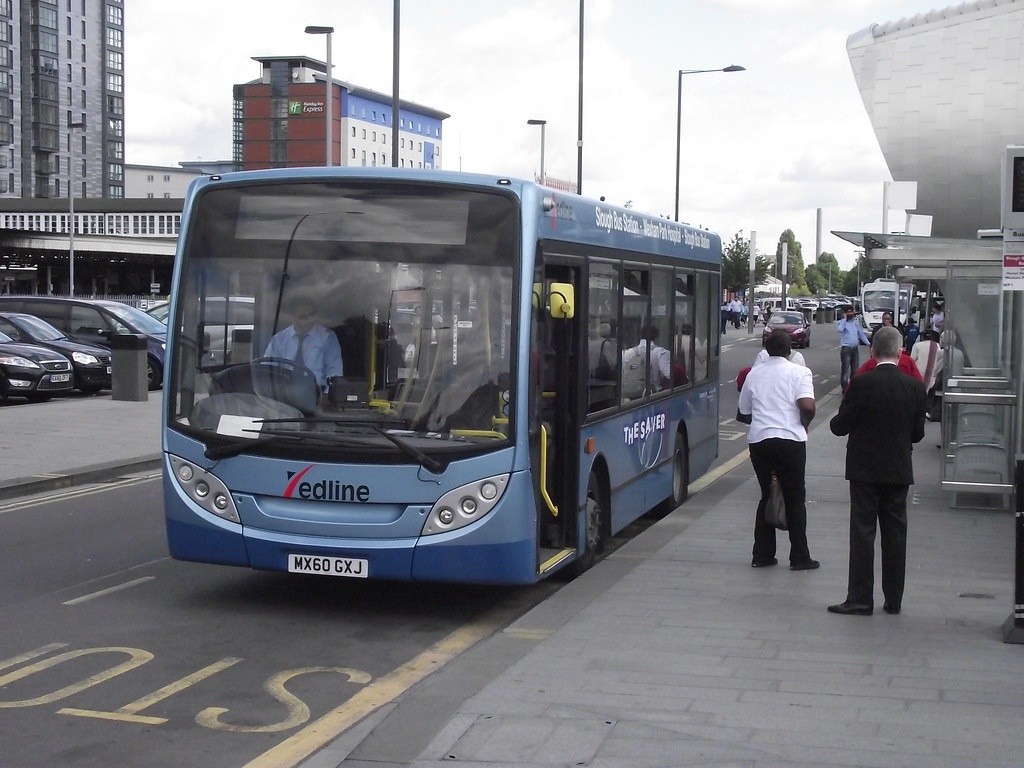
[622,346,656,397]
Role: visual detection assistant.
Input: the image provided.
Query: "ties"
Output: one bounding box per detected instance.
[294,333,309,375]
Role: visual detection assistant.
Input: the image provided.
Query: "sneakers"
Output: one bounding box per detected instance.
[752,558,778,566]
[791,559,819,570]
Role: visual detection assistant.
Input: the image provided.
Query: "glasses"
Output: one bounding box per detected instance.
[295,312,315,320]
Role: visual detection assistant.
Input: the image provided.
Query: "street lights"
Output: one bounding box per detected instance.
[69,123,88,294]
[675,66,747,222]
[527,120,546,185]
[305,26,334,165]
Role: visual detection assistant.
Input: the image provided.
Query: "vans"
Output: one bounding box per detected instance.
[0,296,167,391]
[117,285,256,370]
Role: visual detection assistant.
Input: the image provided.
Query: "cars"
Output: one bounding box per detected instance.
[738,295,861,320]
[762,311,811,349]
[0,313,113,404]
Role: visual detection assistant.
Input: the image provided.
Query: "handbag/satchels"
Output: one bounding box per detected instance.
[764,471,788,531]
[595,340,610,377]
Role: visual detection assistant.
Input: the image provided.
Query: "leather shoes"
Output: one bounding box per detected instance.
[882,603,901,614]
[828,601,873,615]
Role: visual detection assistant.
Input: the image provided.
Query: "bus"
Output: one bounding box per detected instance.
[161,166,723,593]
[858,278,918,346]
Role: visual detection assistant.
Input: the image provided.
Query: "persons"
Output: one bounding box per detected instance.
[851,354,923,382]
[767,304,772,315]
[871,312,897,340]
[899,302,965,421]
[600,324,705,400]
[262,294,343,394]
[827,326,926,615]
[721,296,761,334]
[836,306,871,394]
[740,329,818,569]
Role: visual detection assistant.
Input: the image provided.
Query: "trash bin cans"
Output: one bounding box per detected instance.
[110,334,148,402]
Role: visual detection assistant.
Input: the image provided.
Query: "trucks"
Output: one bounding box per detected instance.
[762,297,796,322]
